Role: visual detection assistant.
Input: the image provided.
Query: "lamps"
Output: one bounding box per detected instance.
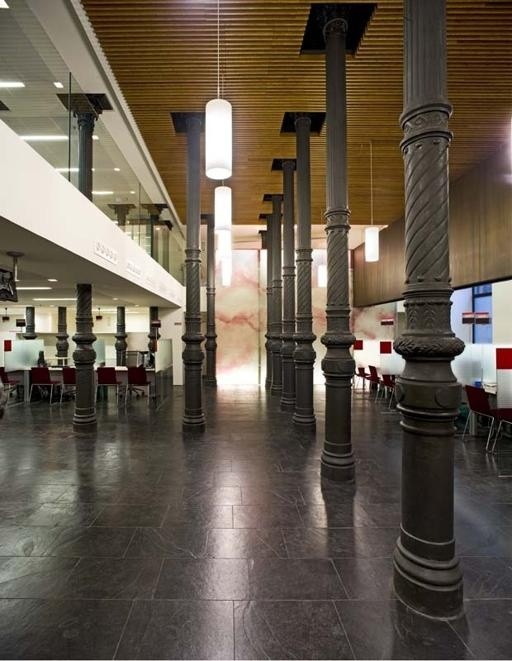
[204,3,233,181]
[317,208,329,289]
[212,76,233,234]
[364,144,380,262]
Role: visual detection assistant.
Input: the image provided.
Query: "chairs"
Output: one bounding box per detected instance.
[0,366,154,407]
[354,365,512,456]
